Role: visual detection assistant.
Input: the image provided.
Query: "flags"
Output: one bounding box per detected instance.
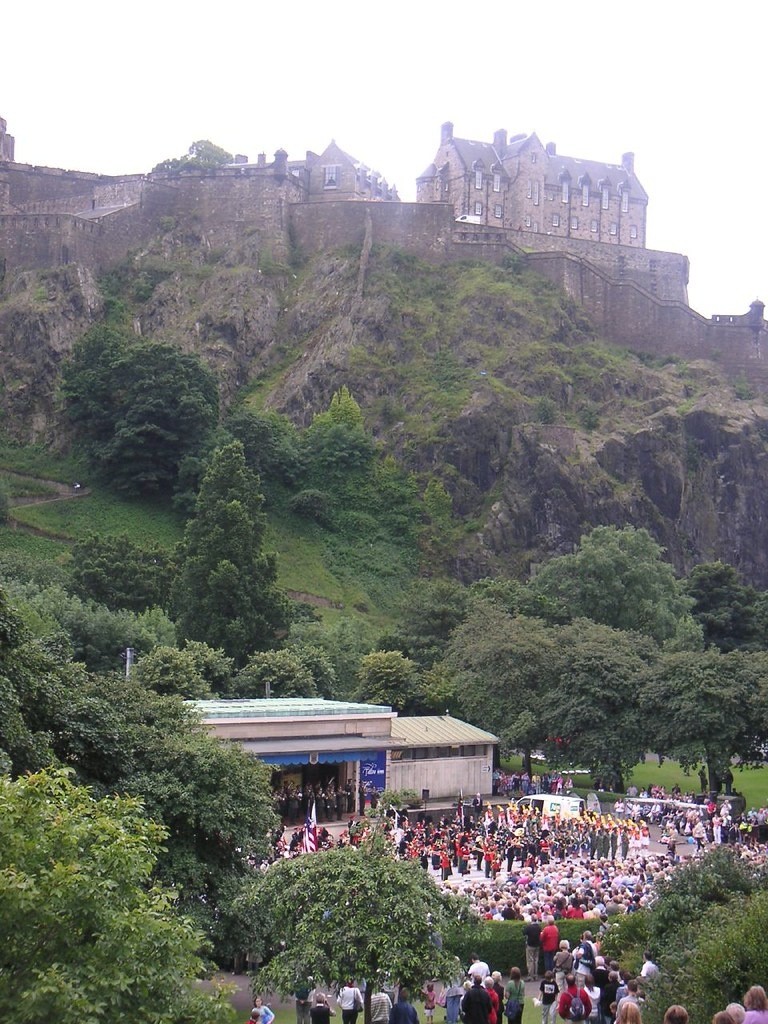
[304,803,315,853]
[458,794,462,818]
[311,803,318,851]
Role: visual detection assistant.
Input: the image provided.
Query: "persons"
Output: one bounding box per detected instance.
[246,913,768,1024]
[247,764,768,923]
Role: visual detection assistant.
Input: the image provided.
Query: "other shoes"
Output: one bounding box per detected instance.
[534,976,538,981]
[524,977,533,982]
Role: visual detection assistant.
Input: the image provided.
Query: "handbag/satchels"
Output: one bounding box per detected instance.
[504,1000,520,1019]
[353,988,362,1011]
[553,965,560,973]
[436,991,447,1008]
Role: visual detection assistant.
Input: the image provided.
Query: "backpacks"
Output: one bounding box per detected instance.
[565,987,585,1021]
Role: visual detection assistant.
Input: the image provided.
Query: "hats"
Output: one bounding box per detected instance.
[273,779,377,803]
[383,800,648,862]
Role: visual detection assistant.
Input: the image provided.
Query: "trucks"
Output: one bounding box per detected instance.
[512,792,585,820]
